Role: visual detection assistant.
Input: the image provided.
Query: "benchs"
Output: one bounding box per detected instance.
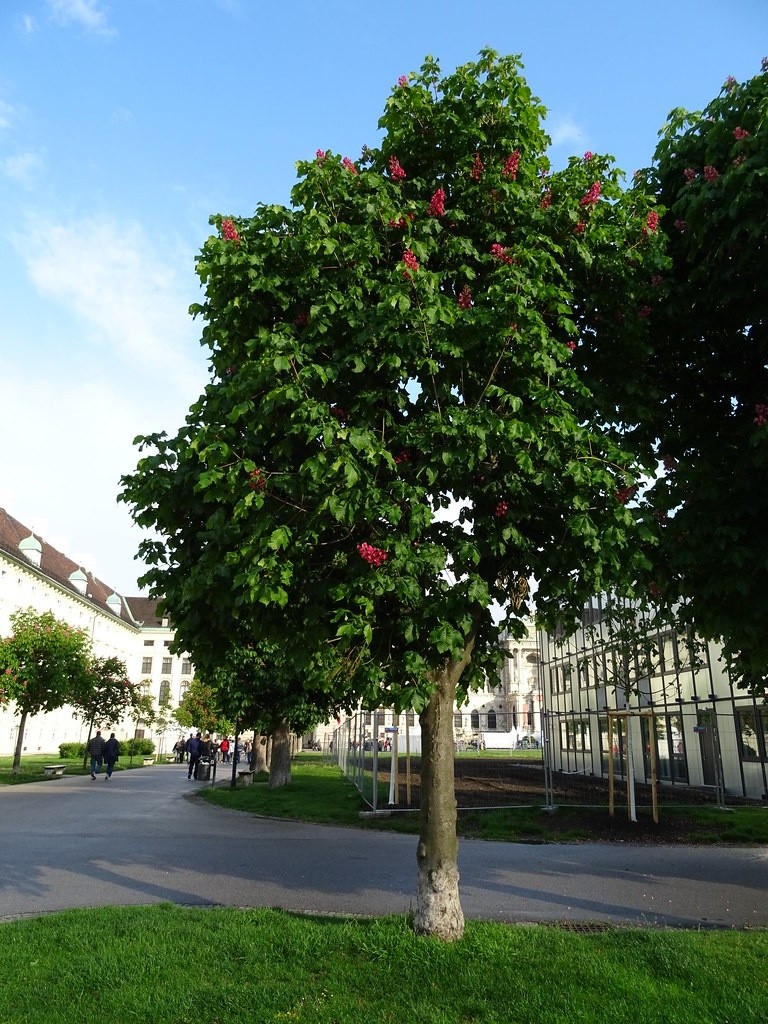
[238,769,255,786]
[167,756,175,763]
[143,758,154,765]
[43,764,66,776]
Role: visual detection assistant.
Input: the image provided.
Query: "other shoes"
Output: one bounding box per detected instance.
[105,774,110,780]
[91,773,96,779]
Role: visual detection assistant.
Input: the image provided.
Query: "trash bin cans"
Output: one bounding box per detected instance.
[197,756,211,782]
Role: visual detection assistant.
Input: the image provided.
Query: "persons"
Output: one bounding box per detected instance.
[307,738,333,753]
[614,745,619,758]
[678,742,682,752]
[512,741,539,750]
[172,732,254,779]
[350,737,392,752]
[87,731,121,781]
